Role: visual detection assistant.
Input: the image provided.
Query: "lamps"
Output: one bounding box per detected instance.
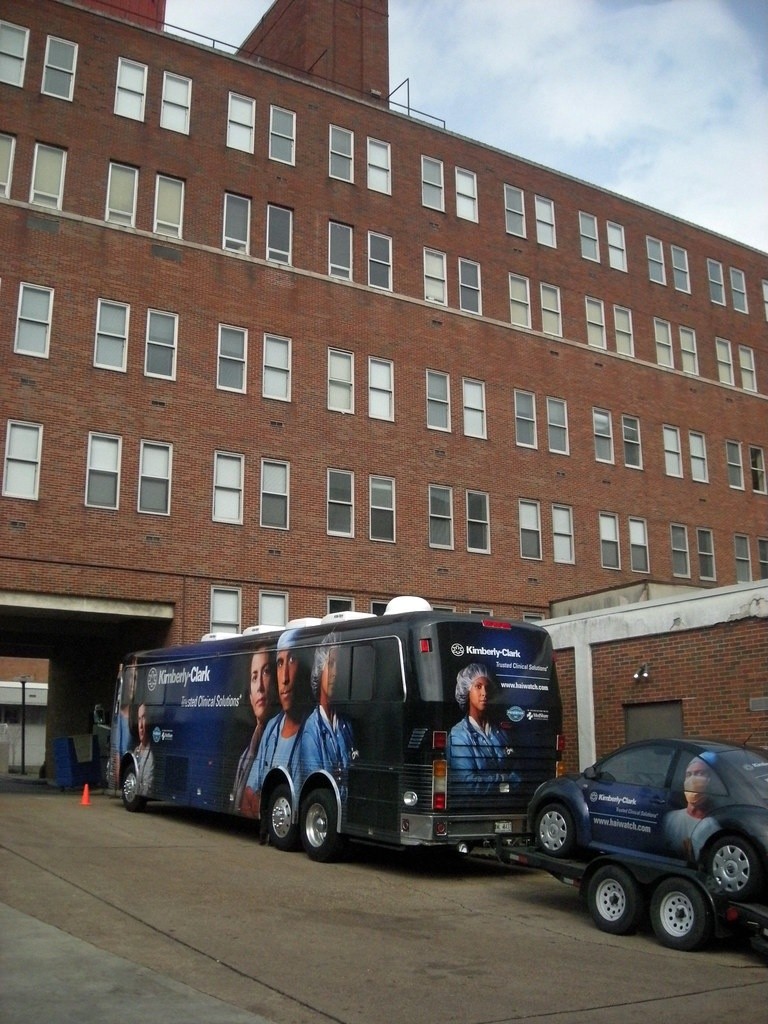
[634,663,648,679]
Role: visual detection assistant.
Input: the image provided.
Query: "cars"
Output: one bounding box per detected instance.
[528,738,768,903]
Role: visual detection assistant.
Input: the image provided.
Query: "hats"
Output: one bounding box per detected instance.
[276,629,314,669]
[698,750,720,773]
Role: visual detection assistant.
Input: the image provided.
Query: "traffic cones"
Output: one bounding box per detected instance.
[79,783,92,805]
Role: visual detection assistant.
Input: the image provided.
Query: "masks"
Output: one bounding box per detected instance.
[684,777,712,803]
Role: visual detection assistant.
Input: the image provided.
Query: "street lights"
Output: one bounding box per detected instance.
[20,675,27,775]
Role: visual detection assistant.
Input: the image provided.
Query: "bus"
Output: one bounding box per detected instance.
[93,596,564,863]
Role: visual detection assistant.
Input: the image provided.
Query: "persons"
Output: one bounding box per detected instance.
[228,628,356,818]
[134,701,155,797]
[114,658,138,783]
[445,663,521,795]
[663,751,723,858]
